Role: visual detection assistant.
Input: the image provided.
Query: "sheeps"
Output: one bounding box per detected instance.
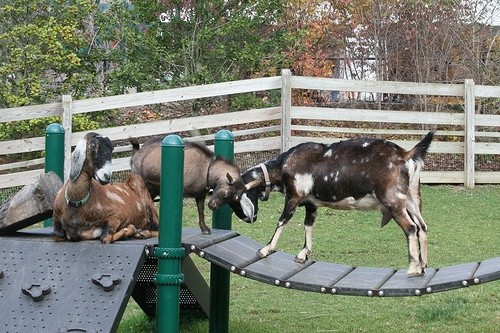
[129,135,261,235]
[49,132,160,244]
[226,127,437,278]
